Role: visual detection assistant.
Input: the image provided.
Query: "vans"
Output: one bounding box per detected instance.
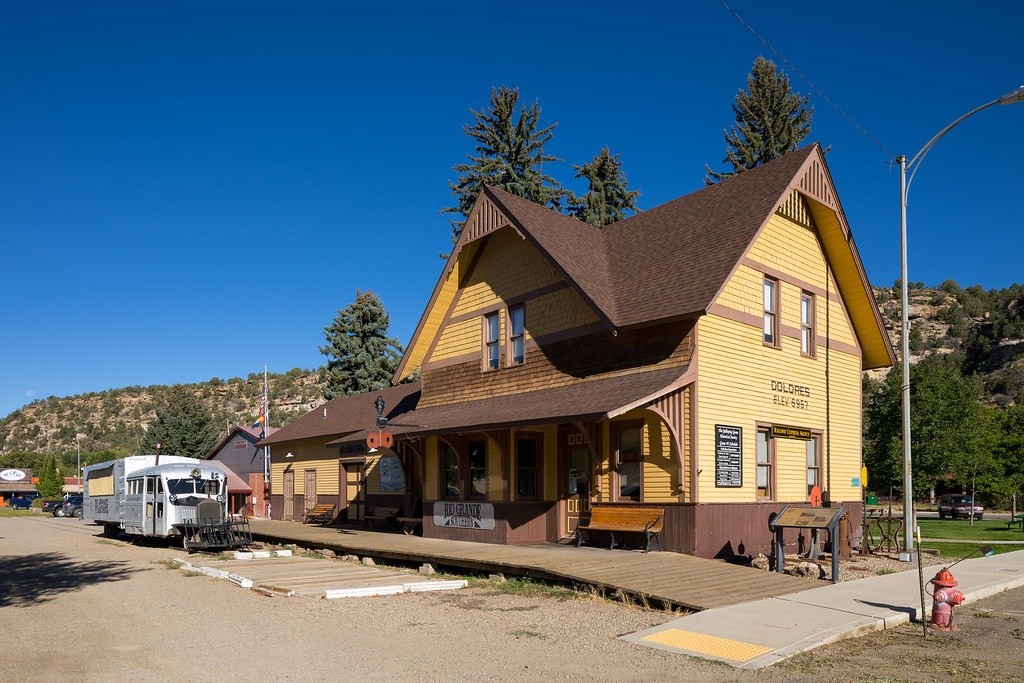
[84,444,253,552]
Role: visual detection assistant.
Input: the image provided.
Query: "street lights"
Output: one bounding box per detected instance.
[896,84,1024,553]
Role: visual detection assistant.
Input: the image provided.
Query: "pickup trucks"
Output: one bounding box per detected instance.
[42,498,69,517]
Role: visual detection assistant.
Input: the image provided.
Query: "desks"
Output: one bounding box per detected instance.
[1014,514,1024,532]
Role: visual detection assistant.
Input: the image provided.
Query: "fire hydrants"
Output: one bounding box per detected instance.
[930,567,966,630]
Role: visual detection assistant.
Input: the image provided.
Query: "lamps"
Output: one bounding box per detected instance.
[285,452,298,457]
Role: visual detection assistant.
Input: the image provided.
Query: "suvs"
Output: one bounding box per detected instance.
[62,494,83,518]
[938,493,985,520]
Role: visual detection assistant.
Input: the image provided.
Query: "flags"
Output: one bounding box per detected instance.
[253,377,268,432]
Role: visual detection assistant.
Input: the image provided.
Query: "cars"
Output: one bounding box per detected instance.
[10,490,42,511]
[442,459,643,500]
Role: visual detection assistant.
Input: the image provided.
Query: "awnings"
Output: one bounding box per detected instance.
[323,364,703,449]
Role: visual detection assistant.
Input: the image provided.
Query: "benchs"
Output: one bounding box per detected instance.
[1004,520,1023,531]
[361,505,401,531]
[302,503,336,527]
[577,507,664,553]
